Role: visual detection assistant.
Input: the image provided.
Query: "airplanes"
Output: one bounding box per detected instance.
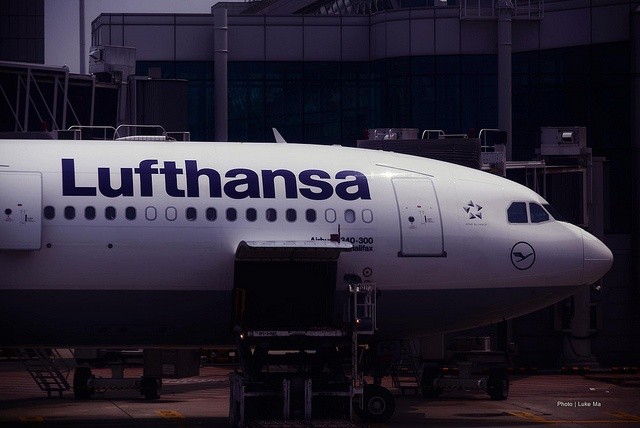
[0,126,615,400]
